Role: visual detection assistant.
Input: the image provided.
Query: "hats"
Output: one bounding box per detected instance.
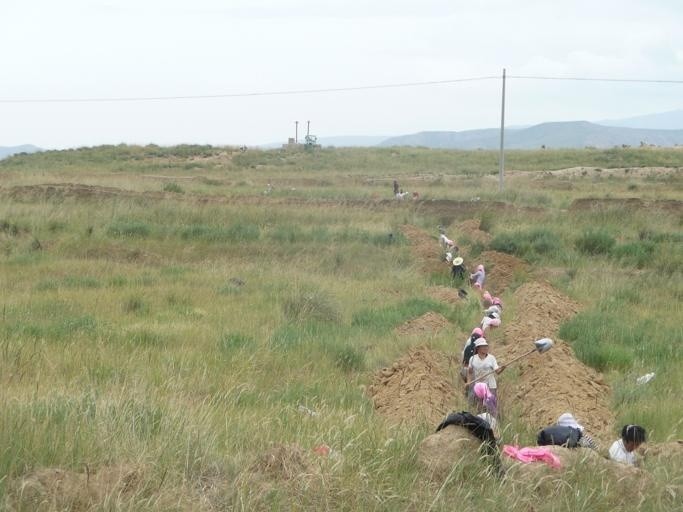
[473,337,489,348]
[453,256,464,266]
[472,327,484,337]
[473,383,490,398]
[483,292,501,305]
[489,319,501,327]
[557,412,588,433]
[477,264,484,272]
[474,282,482,290]
[484,306,500,318]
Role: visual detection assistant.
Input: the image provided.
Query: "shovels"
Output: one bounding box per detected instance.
[465,337,553,387]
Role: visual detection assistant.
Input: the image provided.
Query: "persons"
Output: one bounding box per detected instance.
[557,412,599,453]
[393,180,398,196]
[395,189,408,200]
[411,192,419,201]
[607,423,649,469]
[436,225,503,446]
